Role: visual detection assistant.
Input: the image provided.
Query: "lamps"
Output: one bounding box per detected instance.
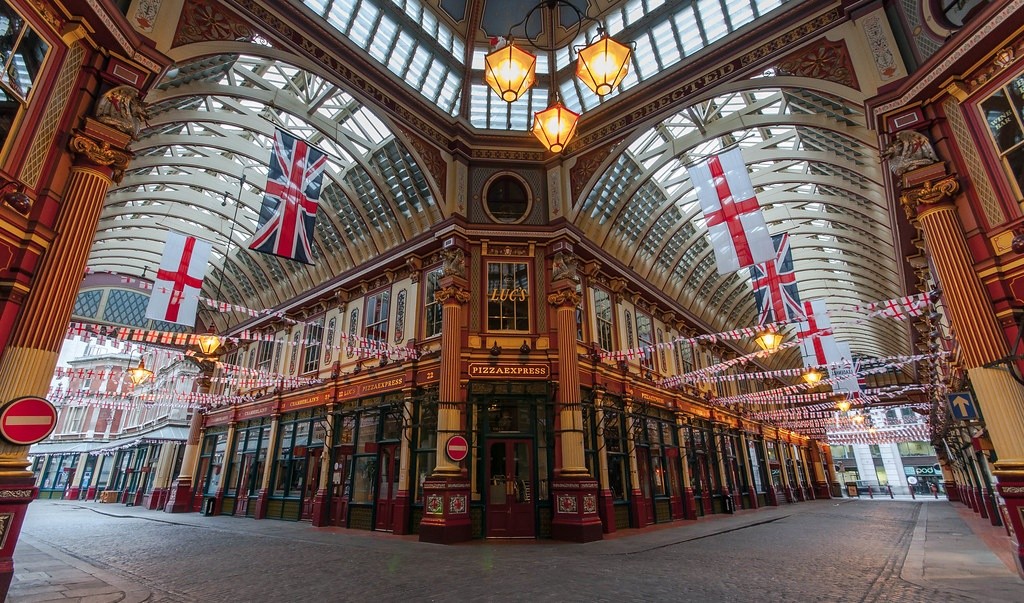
[193,174,247,355]
[751,264,786,353]
[481,0,638,154]
[126,344,158,386]
[796,323,823,387]
[831,370,851,412]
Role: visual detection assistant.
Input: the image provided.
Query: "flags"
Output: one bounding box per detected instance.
[686,147,777,276]
[248,127,328,266]
[599,291,950,444]
[145,231,211,328]
[47,267,417,410]
[749,231,866,400]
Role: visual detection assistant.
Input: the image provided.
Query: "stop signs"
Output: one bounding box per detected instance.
[0,396,58,445]
[446,435,468,462]
